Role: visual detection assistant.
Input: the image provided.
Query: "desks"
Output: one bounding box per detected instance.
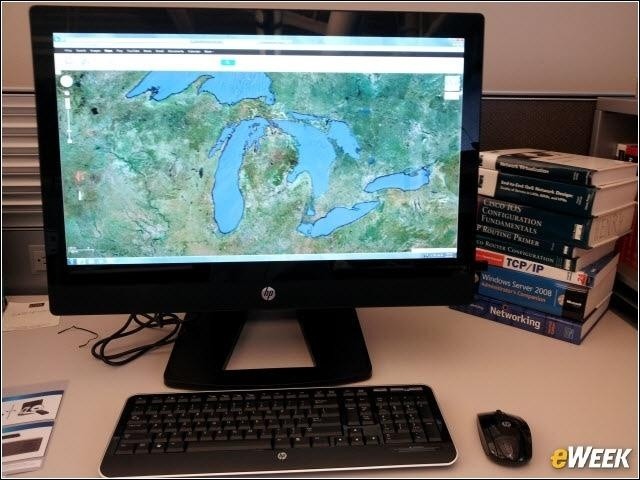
[1,288,640,479]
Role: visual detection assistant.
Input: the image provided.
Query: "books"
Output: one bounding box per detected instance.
[446,147,639,344]
[0,389,64,475]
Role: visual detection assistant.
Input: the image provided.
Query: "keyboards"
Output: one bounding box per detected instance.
[99,383,457,475]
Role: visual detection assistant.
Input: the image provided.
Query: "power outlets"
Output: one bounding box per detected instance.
[27,247,49,275]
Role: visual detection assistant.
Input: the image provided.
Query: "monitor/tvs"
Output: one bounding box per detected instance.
[27,4,487,390]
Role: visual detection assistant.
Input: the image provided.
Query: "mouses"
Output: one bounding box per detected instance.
[478,411,533,464]
